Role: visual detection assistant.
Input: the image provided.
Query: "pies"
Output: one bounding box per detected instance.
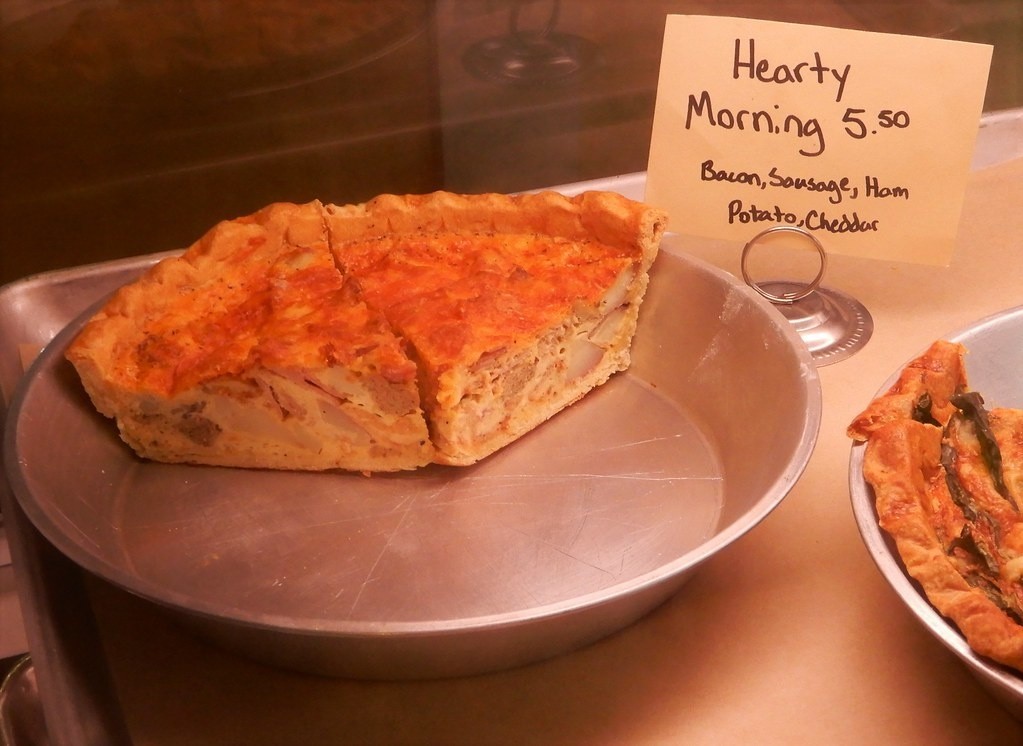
[63,189,670,473]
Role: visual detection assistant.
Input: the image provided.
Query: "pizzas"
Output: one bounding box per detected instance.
[844,338,1022,678]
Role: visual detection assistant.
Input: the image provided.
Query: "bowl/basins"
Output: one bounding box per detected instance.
[2,244,824,682]
[849,307,1023,726]
